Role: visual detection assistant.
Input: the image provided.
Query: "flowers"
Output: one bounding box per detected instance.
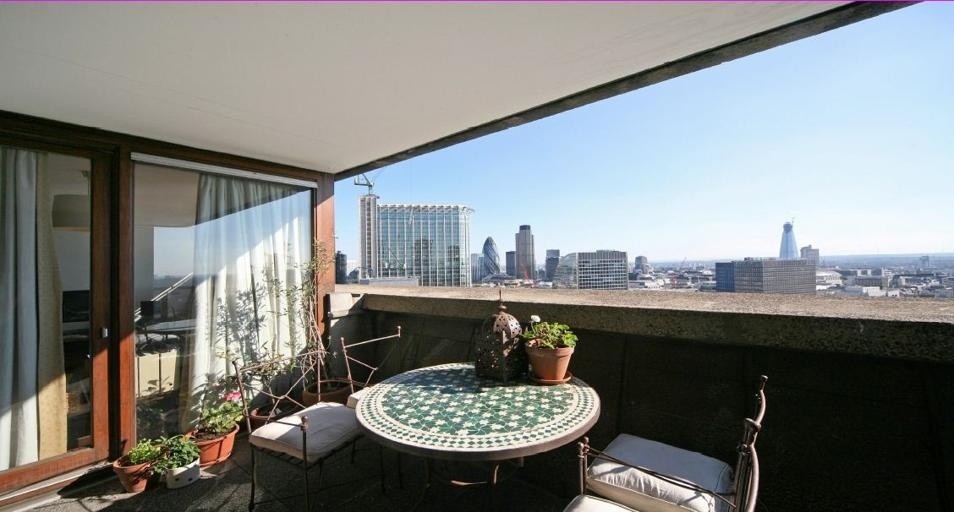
[202,390,244,437]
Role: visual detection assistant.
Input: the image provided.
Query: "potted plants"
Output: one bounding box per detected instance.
[112,435,202,492]
[216,234,356,426]
[523,314,578,383]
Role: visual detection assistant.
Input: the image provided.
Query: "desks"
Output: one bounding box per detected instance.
[356,362,603,511]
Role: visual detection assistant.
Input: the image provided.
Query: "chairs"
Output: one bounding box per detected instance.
[564,373,769,511]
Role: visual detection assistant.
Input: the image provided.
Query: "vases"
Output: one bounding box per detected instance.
[184,422,240,466]
